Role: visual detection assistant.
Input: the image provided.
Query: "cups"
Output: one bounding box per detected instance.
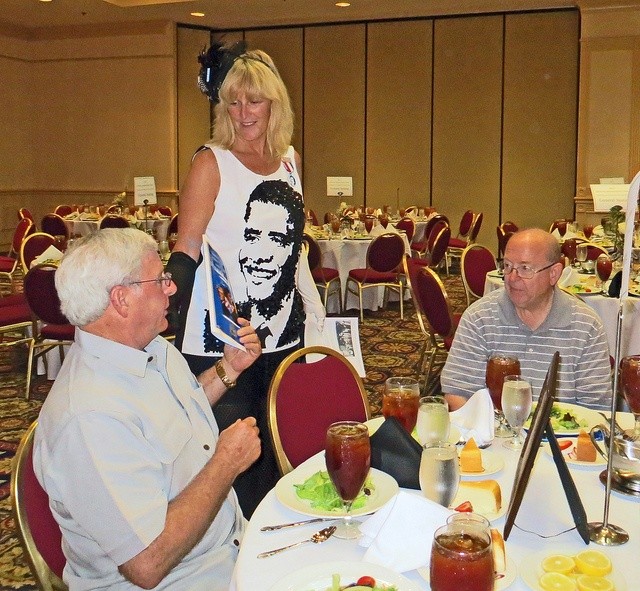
[589,424,639,493]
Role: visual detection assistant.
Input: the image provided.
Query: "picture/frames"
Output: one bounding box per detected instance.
[502,351,561,541]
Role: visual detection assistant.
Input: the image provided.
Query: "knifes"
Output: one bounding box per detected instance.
[260,518,338,531]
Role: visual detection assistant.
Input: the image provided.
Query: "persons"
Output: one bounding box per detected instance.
[32,227,263,590]
[203,180,304,354]
[165,51,305,519]
[440,227,613,413]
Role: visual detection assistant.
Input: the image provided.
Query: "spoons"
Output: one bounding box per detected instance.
[257,526,336,560]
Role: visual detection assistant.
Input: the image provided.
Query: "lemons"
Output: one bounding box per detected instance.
[566,557,583,580]
[542,555,575,574]
[578,573,614,591]
[575,550,612,576]
[540,571,574,591]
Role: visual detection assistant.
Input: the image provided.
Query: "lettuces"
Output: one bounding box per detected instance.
[551,402,588,431]
[292,469,376,512]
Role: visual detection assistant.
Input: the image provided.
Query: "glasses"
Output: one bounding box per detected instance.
[497,260,556,280]
[126,273,173,290]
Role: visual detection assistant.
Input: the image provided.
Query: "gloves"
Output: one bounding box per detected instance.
[162,251,197,334]
[295,244,327,331]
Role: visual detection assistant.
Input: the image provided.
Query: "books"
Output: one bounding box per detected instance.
[202,233,246,352]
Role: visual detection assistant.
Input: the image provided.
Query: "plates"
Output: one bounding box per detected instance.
[522,401,606,435]
[490,495,508,523]
[274,463,399,520]
[458,448,504,477]
[544,437,609,467]
[270,557,427,591]
[576,283,601,294]
[519,548,640,591]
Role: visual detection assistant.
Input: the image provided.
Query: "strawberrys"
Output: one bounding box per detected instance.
[455,501,472,513]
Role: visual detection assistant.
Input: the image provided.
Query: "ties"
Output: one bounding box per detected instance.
[256,322,272,348]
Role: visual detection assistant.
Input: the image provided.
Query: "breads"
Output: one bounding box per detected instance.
[460,436,484,472]
[456,479,502,514]
[490,528,506,575]
[576,428,596,462]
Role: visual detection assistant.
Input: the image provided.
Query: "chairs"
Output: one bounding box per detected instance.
[8,421,65,591]
[17,207,33,225]
[40,214,70,240]
[402,254,461,383]
[20,233,59,273]
[448,211,476,267]
[24,259,75,400]
[448,213,484,268]
[158,206,172,217]
[416,267,454,398]
[0,294,40,377]
[500,233,513,256]
[392,221,451,290]
[0,218,33,294]
[394,218,415,246]
[410,215,449,260]
[54,205,72,219]
[97,214,130,230]
[302,234,342,317]
[548,220,572,238]
[405,206,416,213]
[267,347,370,476]
[406,222,450,267]
[461,244,498,308]
[345,233,405,322]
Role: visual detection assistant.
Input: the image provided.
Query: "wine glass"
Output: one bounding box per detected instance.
[558,221,567,236]
[383,376,421,437]
[423,207,431,220]
[399,207,405,220]
[325,420,372,540]
[583,224,592,240]
[446,512,492,538]
[619,355,640,442]
[416,395,450,450]
[159,239,169,264]
[418,440,460,510]
[429,521,495,590]
[380,216,388,230]
[367,208,374,217]
[500,374,533,453]
[486,355,522,439]
[365,218,373,239]
[332,221,340,236]
[597,258,613,295]
[575,244,588,270]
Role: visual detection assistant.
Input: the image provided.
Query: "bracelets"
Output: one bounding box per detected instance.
[215,359,237,392]
